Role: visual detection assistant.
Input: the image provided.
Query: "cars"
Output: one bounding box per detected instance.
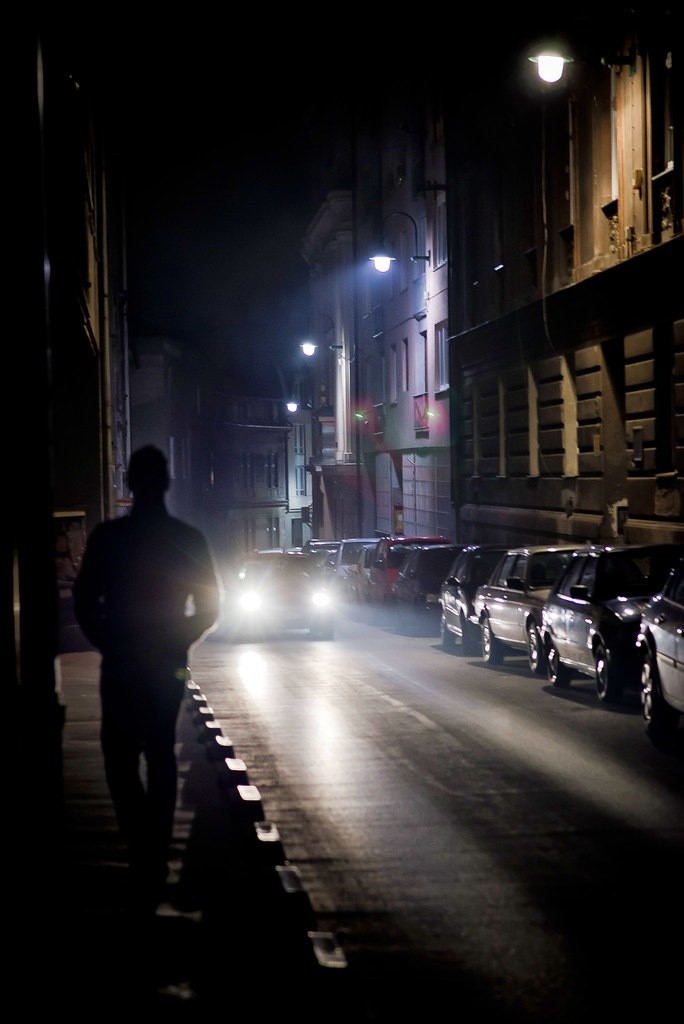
[228,537,379,644]
[636,558,684,740]
[371,535,456,604]
[396,543,474,631]
[540,543,684,705]
[474,545,603,678]
[438,541,525,653]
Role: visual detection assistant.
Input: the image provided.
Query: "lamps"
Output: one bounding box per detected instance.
[368,211,431,272]
[299,312,343,356]
[526,0,637,83]
[285,383,314,412]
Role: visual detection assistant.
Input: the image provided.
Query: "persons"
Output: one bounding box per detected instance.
[662,188,672,228]
[610,215,617,241]
[70,442,218,880]
